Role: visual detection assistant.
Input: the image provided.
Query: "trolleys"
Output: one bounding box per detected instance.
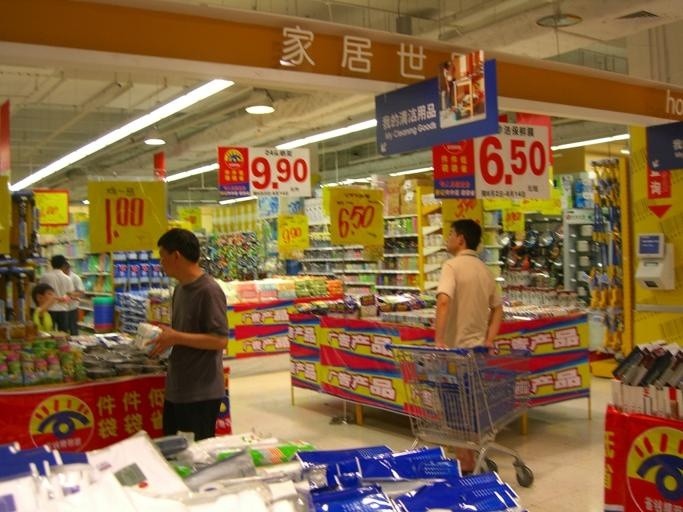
[386,342,536,488]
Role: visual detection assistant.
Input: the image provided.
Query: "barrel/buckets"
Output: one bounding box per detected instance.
[92,296,114,333]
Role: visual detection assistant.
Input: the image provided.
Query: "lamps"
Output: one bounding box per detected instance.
[389,132,631,178]
[245,90,277,115]
[219,176,373,206]
[164,118,379,182]
[7,77,235,192]
[143,127,167,146]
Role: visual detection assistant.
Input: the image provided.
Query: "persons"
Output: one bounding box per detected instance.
[38,254,74,332]
[62,261,85,335]
[31,284,58,331]
[144,228,228,443]
[434,218,503,475]
